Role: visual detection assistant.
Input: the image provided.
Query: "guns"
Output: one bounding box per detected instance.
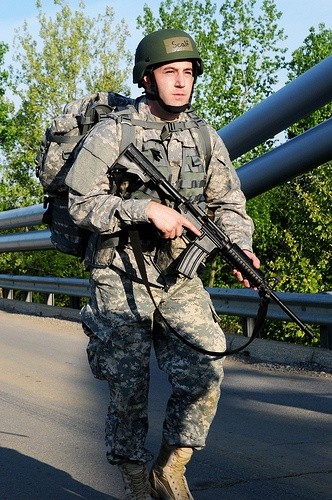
[104,142,316,341]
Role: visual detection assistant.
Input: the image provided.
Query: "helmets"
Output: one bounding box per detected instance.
[132,28,205,83]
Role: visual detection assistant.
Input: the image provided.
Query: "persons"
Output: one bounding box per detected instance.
[63,28,260,500]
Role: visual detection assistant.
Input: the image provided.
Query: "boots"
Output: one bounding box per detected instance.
[115,462,155,500]
[150,439,198,500]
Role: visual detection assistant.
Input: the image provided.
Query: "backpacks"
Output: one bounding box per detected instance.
[36,91,212,256]
